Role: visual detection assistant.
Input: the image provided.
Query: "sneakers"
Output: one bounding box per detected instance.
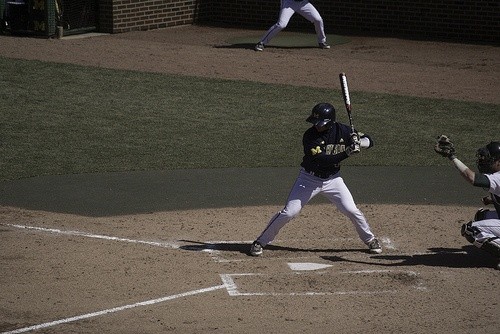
[369,241,382,254]
[250,241,262,256]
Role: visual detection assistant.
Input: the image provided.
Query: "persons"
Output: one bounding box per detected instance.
[255,0,330,52]
[250,102,383,257]
[433,134,500,269]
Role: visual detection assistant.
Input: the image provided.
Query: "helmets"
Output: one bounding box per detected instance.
[487,141,500,159]
[305,102,336,128]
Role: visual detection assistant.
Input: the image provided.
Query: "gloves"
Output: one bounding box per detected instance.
[345,144,360,155]
[350,132,361,145]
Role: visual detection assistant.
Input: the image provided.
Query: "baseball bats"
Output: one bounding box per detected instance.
[339,72,361,154]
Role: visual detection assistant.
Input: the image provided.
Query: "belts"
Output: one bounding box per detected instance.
[305,168,330,179]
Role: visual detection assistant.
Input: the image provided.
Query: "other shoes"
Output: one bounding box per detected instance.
[257,43,263,52]
[320,42,331,49]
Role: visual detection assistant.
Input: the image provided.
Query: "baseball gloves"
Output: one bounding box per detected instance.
[434,134,456,160]
